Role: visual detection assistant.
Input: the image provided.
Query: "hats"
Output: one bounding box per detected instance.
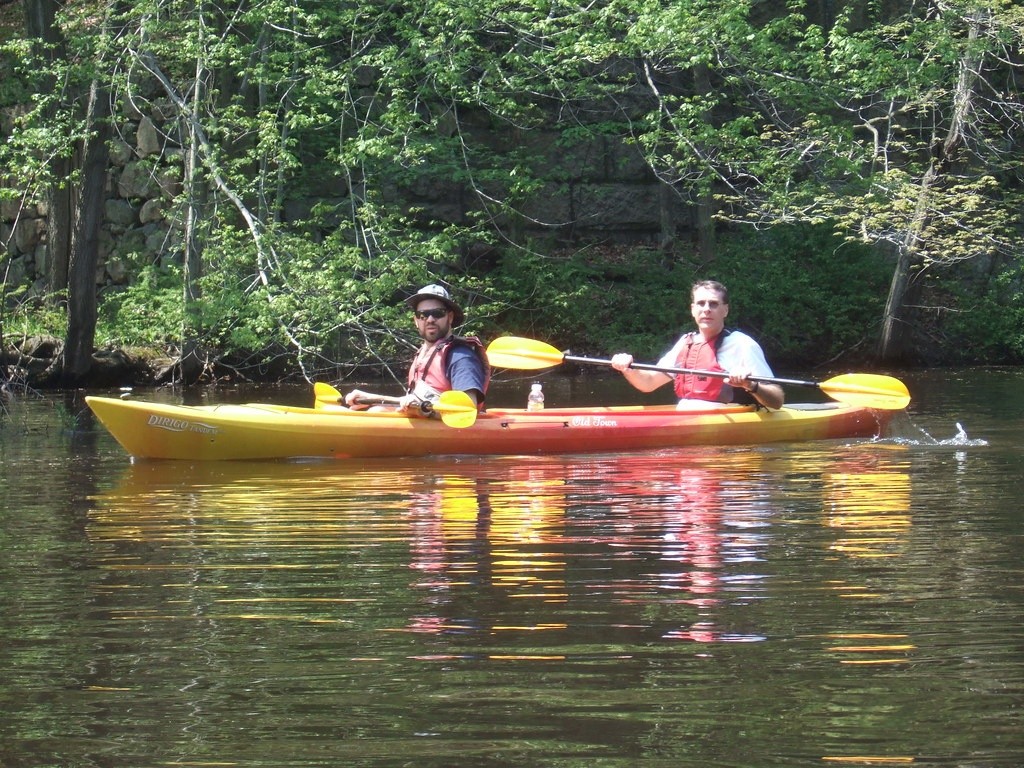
[404,284,464,328]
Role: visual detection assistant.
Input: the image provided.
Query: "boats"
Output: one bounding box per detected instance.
[82,394,906,460]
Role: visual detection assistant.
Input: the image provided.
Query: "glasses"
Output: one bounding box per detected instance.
[413,308,447,318]
[691,299,724,310]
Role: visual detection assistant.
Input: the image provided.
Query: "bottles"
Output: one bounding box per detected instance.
[526,384,545,411]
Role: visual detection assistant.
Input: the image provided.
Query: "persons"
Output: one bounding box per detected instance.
[610,279,785,412]
[344,283,486,418]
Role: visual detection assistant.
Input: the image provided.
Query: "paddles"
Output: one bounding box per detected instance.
[481,328,914,415]
[309,378,482,433]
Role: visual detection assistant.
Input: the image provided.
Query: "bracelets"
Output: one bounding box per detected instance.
[744,381,759,393]
